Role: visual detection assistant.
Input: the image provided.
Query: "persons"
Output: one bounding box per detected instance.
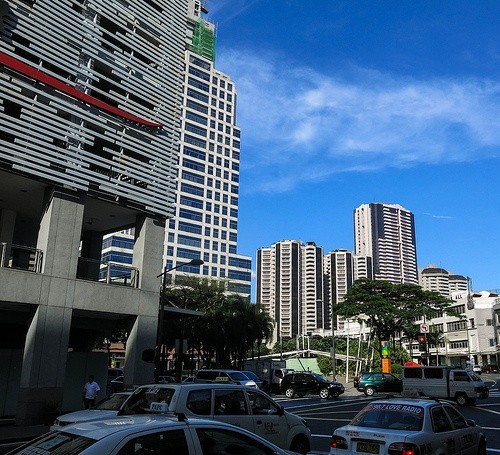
[83,375,100,409]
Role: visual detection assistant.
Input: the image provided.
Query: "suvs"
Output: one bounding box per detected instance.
[280,370,346,400]
[352,371,403,397]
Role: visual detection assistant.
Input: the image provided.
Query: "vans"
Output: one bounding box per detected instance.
[242,369,263,392]
[193,368,260,391]
[116,375,313,455]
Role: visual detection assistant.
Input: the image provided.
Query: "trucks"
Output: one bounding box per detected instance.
[401,365,490,406]
[242,356,295,395]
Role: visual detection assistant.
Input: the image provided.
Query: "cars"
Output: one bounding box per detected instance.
[182,375,197,385]
[158,375,176,384]
[328,391,487,455]
[111,375,125,393]
[50,384,144,432]
[2,400,305,455]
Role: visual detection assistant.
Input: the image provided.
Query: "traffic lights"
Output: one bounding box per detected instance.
[418,334,426,353]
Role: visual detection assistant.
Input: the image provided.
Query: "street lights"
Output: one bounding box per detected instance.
[315,297,337,382]
